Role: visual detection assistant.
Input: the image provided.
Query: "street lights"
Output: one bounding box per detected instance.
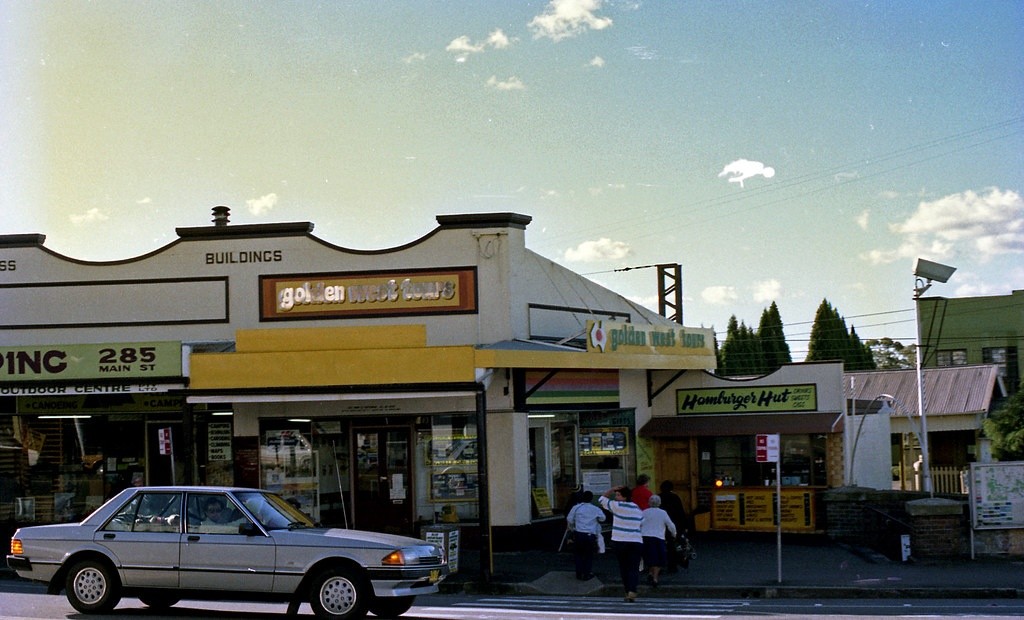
[914,257,957,493]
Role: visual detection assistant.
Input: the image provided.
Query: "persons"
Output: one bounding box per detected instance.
[231,494,249,521]
[198,498,262,534]
[598,485,644,602]
[566,491,606,581]
[658,480,690,572]
[642,494,677,585]
[629,473,655,508]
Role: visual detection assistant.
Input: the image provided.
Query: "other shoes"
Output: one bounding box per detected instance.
[646,575,653,585]
[639,566,645,572]
[583,571,595,581]
[652,581,659,588]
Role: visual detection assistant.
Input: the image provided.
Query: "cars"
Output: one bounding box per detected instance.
[9,485,456,620]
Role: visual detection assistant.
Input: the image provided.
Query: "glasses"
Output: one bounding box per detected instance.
[207,508,222,513]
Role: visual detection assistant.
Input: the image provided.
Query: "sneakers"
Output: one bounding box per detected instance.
[624,591,639,603]
